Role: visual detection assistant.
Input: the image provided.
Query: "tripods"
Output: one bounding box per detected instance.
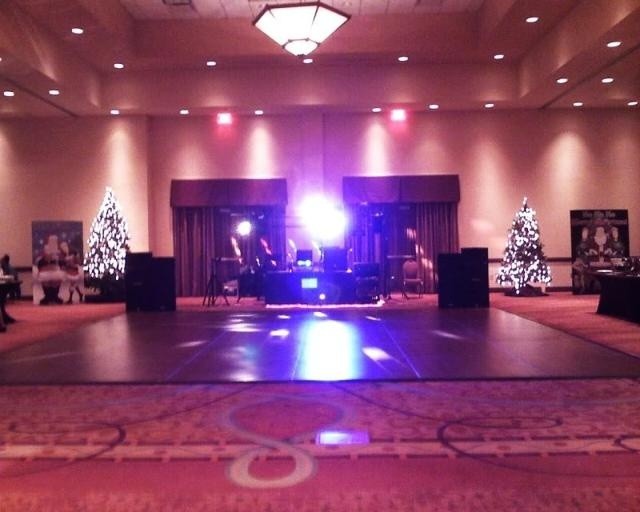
[202,259,229,307]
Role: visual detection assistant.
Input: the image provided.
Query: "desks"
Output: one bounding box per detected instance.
[587,268,640,323]
[0,280,24,333]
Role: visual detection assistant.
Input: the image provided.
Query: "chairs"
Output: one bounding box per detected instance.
[402,259,424,300]
[352,261,381,301]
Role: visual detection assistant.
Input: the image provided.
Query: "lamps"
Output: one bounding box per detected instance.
[252,0,351,58]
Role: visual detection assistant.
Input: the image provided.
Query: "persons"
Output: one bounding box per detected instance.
[59,240,83,303]
[33,235,63,306]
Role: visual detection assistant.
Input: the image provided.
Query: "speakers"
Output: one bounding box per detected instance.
[124,250,176,312]
[436,246,490,309]
